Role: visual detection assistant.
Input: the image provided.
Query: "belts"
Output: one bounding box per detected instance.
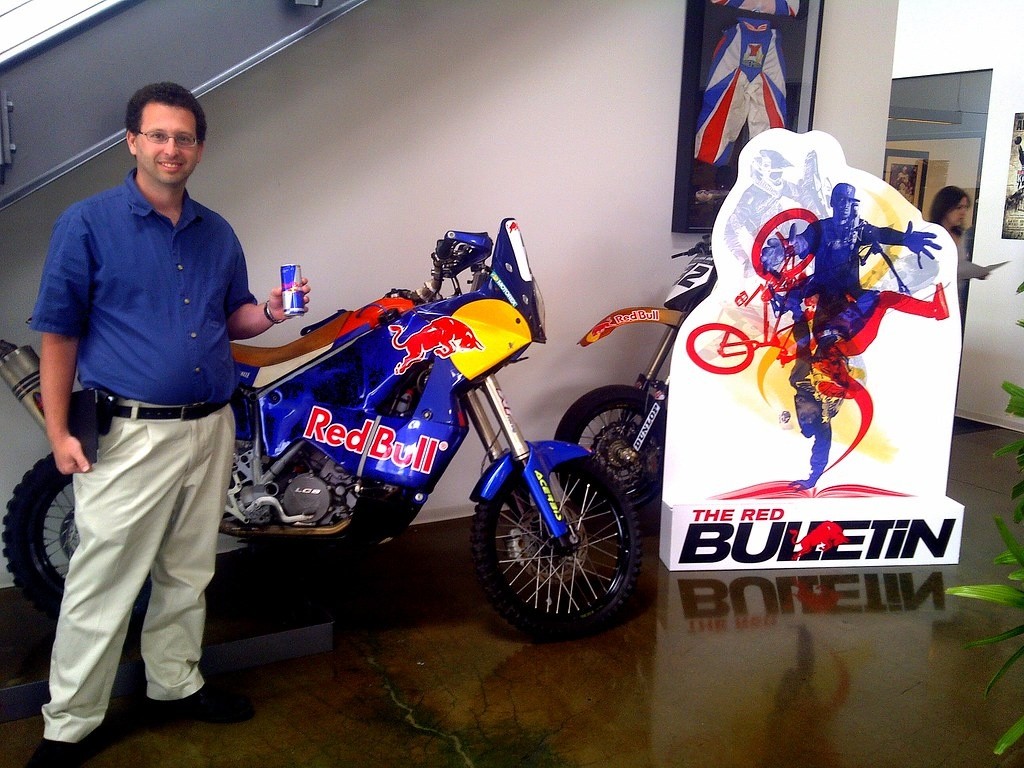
[112,397,229,420]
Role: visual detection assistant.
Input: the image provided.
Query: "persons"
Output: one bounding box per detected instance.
[927,186,989,324]
[25,82,311,768]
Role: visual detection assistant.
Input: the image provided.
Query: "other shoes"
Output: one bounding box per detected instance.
[144,683,255,723]
[25,725,102,768]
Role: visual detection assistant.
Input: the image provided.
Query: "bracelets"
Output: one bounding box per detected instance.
[264,300,285,323]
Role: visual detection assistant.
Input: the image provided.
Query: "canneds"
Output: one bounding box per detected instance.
[279,263,305,315]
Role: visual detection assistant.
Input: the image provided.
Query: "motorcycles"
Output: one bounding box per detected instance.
[551,230,718,517]
[1,216,643,644]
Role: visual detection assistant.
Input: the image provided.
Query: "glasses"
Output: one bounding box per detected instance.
[136,130,201,148]
